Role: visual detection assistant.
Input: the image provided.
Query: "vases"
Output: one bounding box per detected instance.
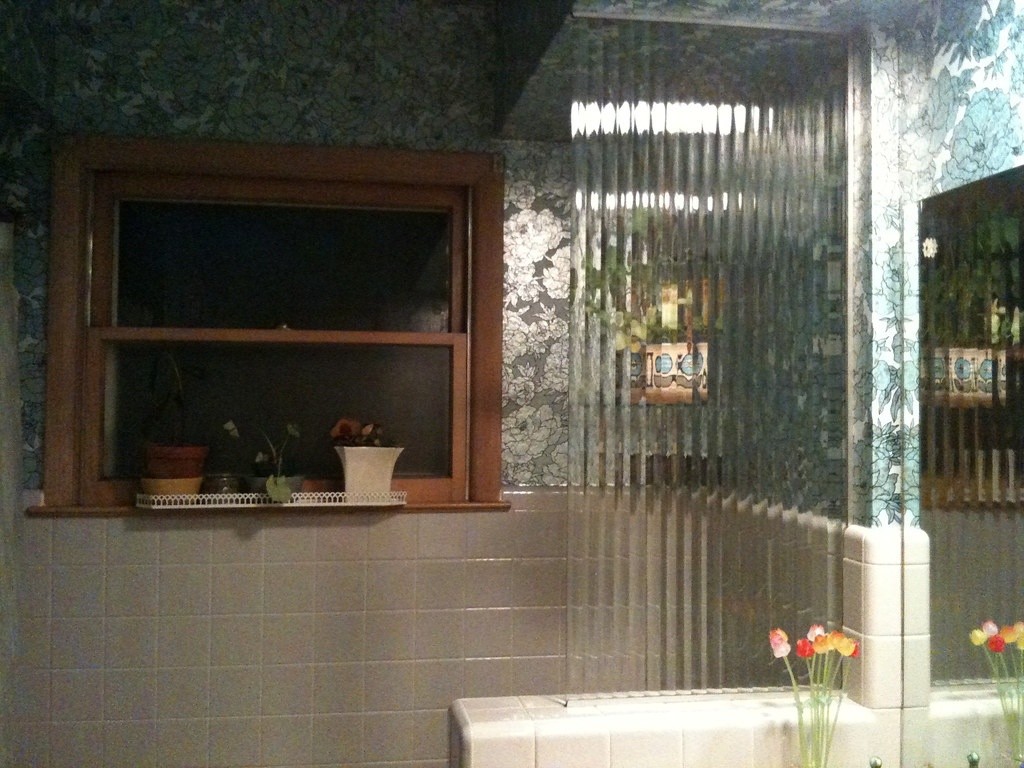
[139,440,209,495]
[204,471,246,496]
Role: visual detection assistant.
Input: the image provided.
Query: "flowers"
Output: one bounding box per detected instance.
[967,619,1022,767]
[767,621,859,767]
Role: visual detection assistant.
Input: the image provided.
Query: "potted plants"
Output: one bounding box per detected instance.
[328,417,403,505]
[223,414,303,503]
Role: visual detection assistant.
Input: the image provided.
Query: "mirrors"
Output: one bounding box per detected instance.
[917,163,1022,767]
